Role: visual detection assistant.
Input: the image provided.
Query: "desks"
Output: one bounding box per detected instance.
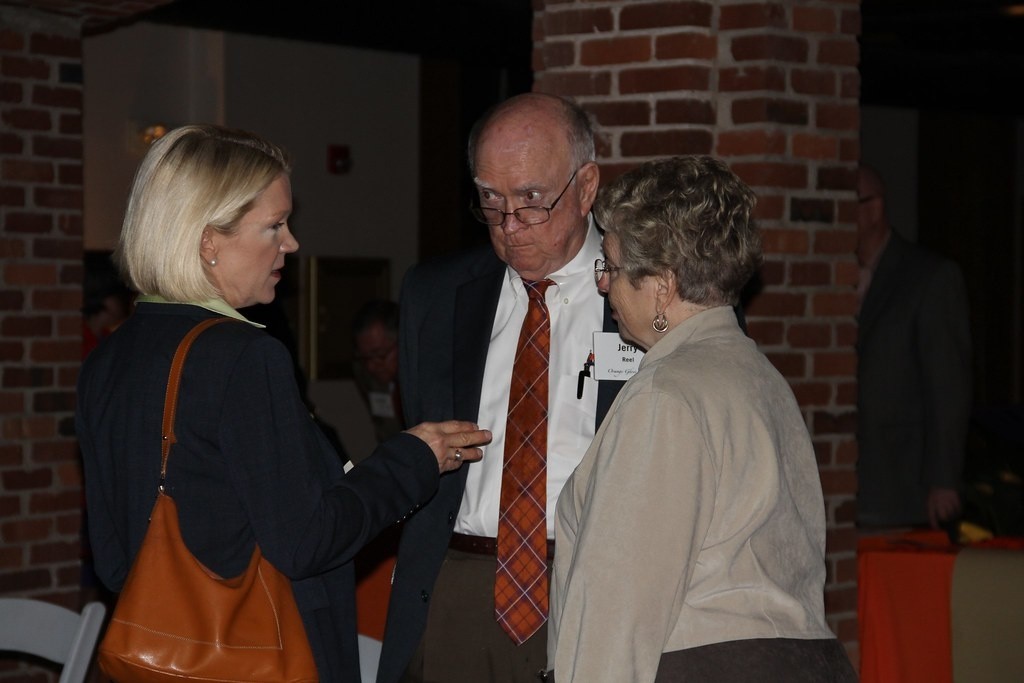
[858,530,1024,683]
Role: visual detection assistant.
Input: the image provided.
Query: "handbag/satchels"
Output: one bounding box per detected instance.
[94,317,321,683]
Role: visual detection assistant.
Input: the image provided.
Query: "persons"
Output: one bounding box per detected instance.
[74,123,493,683]
[373,93,748,683]
[857,165,976,528]
[81,248,134,362]
[545,156,858,683]
[350,294,407,442]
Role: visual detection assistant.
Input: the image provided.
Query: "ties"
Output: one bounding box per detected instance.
[494,278,550,647]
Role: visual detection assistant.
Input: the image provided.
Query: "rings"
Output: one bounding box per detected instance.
[454,449,462,463]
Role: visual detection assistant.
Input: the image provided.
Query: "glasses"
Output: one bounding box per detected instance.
[472,168,577,224]
[355,341,397,361]
